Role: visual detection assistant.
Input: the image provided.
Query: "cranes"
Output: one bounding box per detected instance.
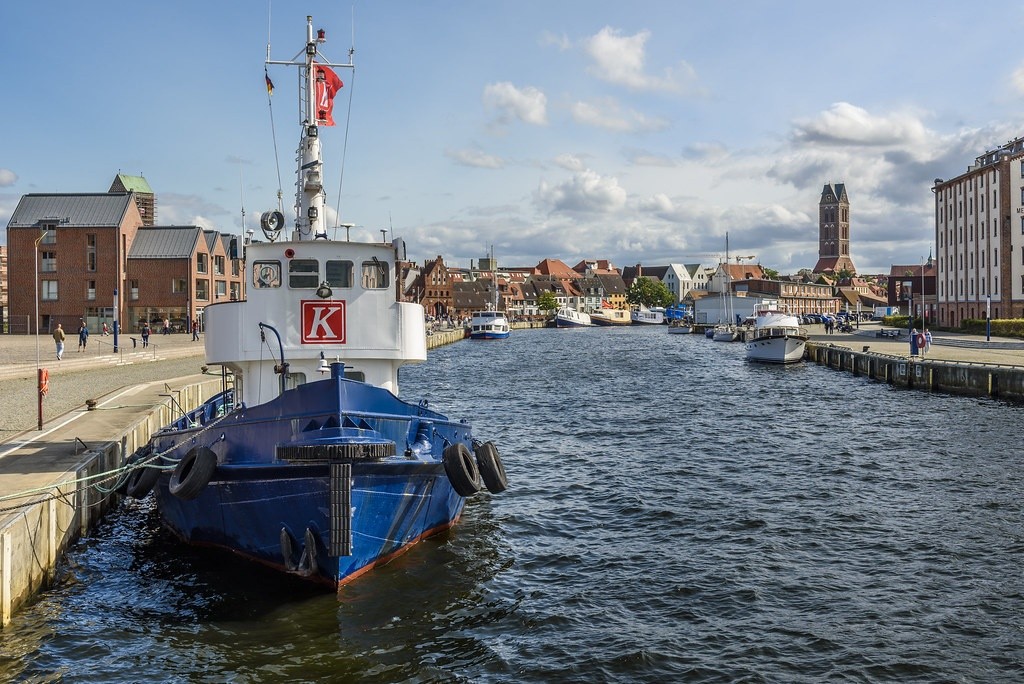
[686,255,755,264]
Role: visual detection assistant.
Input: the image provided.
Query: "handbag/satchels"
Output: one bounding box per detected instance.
[141,339,143,344]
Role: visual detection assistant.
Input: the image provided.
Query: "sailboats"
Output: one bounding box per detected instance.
[704,230,758,343]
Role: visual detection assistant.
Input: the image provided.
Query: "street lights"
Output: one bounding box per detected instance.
[36,231,48,387]
[922,256,937,357]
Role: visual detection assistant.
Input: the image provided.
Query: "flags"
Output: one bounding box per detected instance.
[313,61,342,126]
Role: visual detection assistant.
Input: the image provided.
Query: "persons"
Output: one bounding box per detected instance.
[825,320,851,334]
[924,329,932,354]
[163,318,170,335]
[191,321,199,341]
[101,322,109,337]
[909,328,918,341]
[53,324,65,360]
[77,322,88,352]
[426,314,470,327]
[142,323,149,348]
[426,328,433,335]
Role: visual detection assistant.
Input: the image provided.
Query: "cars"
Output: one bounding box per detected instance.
[798,311,873,332]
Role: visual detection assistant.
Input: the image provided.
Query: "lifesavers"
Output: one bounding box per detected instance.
[40,368,48,395]
[169,445,216,501]
[443,442,481,497]
[126,452,163,500]
[115,446,144,494]
[916,333,926,348]
[476,440,508,494]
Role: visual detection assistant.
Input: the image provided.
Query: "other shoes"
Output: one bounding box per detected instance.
[57,355,61,361]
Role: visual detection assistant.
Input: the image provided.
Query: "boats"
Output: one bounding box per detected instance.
[115,0,507,596]
[743,294,811,365]
[550,303,602,328]
[589,299,632,327]
[468,301,510,340]
[667,306,694,335]
[631,302,667,325]
